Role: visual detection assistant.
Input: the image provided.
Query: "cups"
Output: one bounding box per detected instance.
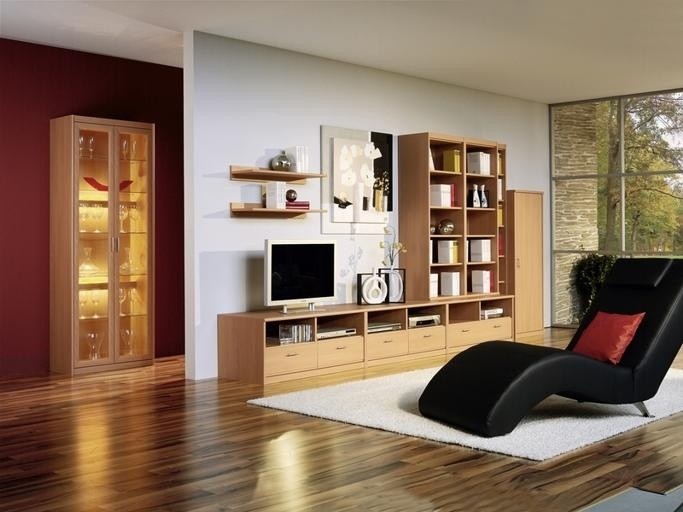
[86,326,105,360]
[121,324,137,358]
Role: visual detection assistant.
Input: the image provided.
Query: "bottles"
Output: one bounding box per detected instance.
[472,183,489,208]
[118,246,143,275]
[78,247,101,276]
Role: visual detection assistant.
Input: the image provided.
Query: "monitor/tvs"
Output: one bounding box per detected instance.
[264,239,338,307]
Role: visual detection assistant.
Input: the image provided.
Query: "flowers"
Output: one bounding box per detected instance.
[379,226,408,266]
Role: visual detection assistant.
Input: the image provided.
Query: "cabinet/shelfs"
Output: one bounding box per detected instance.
[217,295,514,384]
[399,132,498,301]
[230,164,328,214]
[507,190,544,334]
[498,144,507,294]
[49,115,156,377]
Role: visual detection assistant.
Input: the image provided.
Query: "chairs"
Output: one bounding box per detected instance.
[419,258,683,438]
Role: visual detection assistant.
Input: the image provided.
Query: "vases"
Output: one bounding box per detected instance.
[357,267,390,305]
[378,266,406,304]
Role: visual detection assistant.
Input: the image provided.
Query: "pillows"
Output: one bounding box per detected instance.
[571,311,647,366]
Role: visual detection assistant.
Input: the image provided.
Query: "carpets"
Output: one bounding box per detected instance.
[247,367,683,461]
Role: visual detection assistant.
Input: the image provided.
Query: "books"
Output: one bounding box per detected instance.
[265,181,309,211]
[287,145,312,173]
[428,145,505,298]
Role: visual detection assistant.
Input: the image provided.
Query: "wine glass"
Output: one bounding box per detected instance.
[79,134,86,158]
[118,204,128,233]
[78,202,90,233]
[86,135,97,159]
[90,289,104,318]
[120,136,130,161]
[128,204,142,233]
[90,202,104,234]
[79,288,90,319]
[130,136,141,161]
[127,286,140,315]
[119,287,128,316]
